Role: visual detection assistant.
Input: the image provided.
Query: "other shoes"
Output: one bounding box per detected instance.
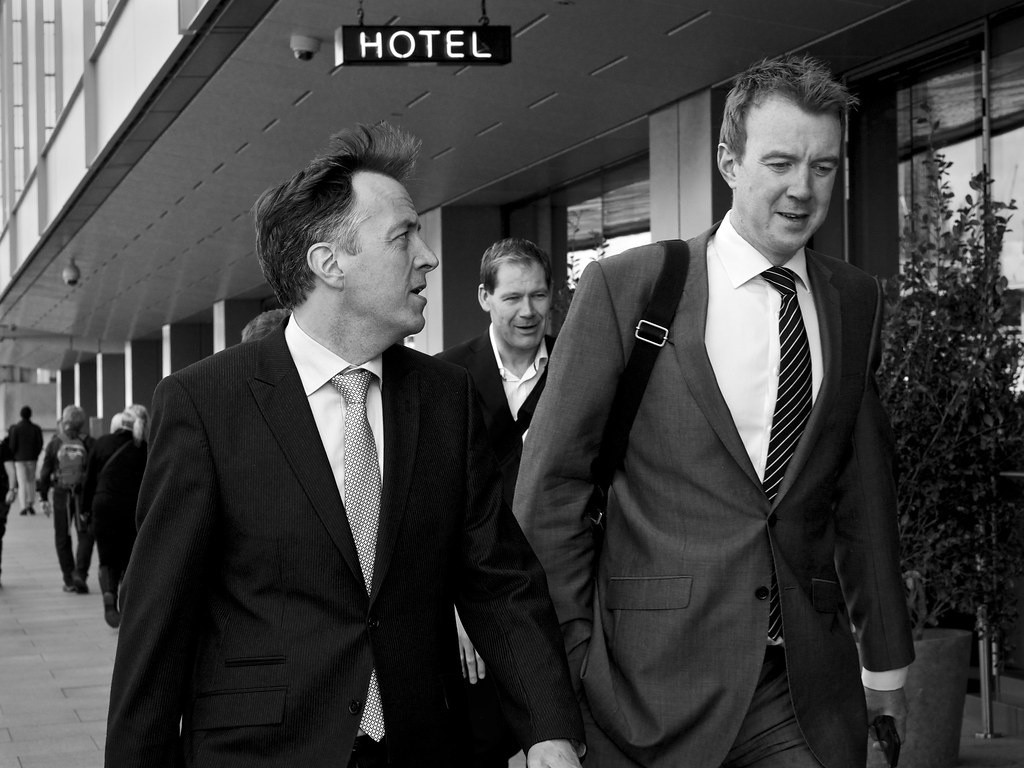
[103,592,120,627]
[62,584,76,592]
[28,506,36,514]
[20,508,27,515]
[75,577,88,593]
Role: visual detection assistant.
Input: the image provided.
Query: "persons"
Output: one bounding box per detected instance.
[428,237,558,768]
[242,308,292,343]
[40,407,93,594]
[510,58,916,767]
[105,125,586,768]
[80,406,148,628]
[0,406,43,585]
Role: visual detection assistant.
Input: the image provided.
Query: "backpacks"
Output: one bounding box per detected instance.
[56,432,88,490]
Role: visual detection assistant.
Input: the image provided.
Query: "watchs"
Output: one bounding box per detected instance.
[9,488,18,493]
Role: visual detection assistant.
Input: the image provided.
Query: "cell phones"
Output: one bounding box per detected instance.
[874,715,901,768]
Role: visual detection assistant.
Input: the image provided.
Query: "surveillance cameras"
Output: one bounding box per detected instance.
[63,265,80,287]
[290,35,321,61]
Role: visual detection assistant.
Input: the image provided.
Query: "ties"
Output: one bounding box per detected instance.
[331,370,387,742]
[757,267,814,641]
